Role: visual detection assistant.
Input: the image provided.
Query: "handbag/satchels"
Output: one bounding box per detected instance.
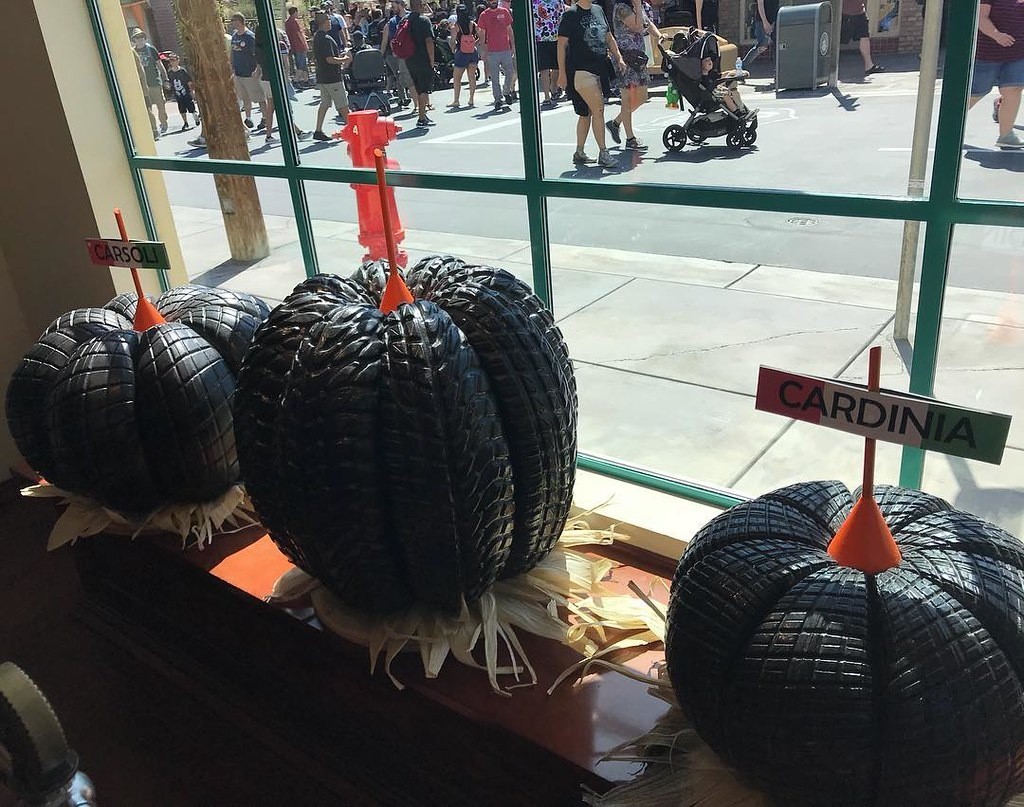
[619,48,649,69]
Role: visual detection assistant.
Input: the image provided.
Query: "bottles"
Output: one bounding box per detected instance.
[735,57,743,78]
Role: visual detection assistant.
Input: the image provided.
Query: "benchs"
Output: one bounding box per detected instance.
[643,26,738,73]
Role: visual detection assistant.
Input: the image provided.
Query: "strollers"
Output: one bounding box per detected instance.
[343,49,391,116]
[657,26,758,152]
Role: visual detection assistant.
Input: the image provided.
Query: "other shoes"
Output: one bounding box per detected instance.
[387,90,437,129]
[614,89,622,97]
[603,97,608,104]
[468,101,473,105]
[484,76,563,110]
[740,108,759,121]
[446,101,460,107]
[293,80,316,89]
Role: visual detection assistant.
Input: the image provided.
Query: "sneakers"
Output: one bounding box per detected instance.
[152,117,333,148]
[992,95,1003,123]
[626,138,649,151]
[995,130,1024,147]
[598,151,619,167]
[605,120,621,144]
[573,152,597,163]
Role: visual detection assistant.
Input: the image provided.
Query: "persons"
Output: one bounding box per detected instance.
[913,0,1024,148]
[841,0,885,74]
[130,0,782,167]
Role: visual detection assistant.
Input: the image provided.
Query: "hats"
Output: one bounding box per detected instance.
[131,28,146,43]
[325,1,333,5]
[321,2,329,9]
[308,6,320,12]
[456,4,467,11]
[440,19,448,25]
[477,4,486,9]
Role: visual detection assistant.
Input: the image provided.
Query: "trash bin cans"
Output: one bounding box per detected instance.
[775,1,833,93]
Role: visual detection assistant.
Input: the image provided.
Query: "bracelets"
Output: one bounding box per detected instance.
[165,79,170,82]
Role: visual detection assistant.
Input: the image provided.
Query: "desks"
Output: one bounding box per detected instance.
[10,458,689,807]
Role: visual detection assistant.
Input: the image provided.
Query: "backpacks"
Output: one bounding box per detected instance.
[457,21,475,53]
[390,15,419,59]
[367,21,380,45]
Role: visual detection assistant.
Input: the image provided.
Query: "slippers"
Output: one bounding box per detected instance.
[865,64,887,74]
